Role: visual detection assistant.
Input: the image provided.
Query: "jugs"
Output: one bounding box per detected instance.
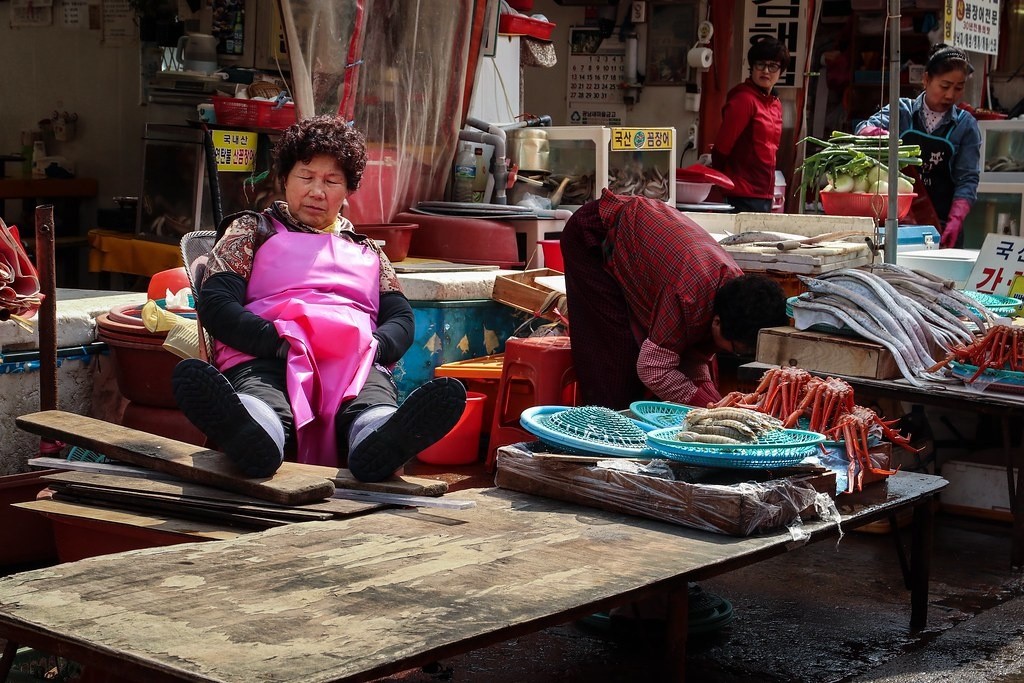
[175,32,218,75]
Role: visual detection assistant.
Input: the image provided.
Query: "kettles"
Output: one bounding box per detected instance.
[507,170,571,210]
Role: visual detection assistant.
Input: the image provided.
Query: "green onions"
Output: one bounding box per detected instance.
[794,131,922,213]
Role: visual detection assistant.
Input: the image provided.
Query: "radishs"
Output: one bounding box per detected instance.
[868,166,888,184]
[823,184,833,192]
[826,173,834,186]
[869,180,888,193]
[853,176,870,192]
[898,177,913,194]
[834,175,854,193]
[854,190,866,193]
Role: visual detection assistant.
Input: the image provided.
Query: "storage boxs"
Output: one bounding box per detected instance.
[538,125,677,214]
[895,248,982,290]
[0,284,149,476]
[739,266,814,317]
[389,296,527,402]
[893,438,935,475]
[505,126,550,172]
[875,222,941,251]
[938,450,1017,511]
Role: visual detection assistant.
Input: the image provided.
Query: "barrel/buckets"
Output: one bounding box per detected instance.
[490,128,553,173]
[769,186,785,214]
[416,392,486,464]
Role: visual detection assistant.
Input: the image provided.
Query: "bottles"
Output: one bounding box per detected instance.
[472,149,487,203]
[234,10,243,54]
[32,141,48,181]
[453,146,476,204]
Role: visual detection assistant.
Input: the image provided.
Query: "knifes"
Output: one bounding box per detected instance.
[776,243,824,250]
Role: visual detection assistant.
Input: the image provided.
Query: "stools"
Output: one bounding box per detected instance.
[486,335,580,473]
[511,219,569,271]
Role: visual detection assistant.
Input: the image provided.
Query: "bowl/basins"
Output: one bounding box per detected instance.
[775,170,787,187]
[147,267,191,303]
[537,240,564,272]
[351,224,419,262]
[41,510,210,566]
[392,212,527,266]
[122,401,205,447]
[100,307,208,407]
[674,181,716,205]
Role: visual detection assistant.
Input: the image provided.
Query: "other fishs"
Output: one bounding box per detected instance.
[716,231,1000,388]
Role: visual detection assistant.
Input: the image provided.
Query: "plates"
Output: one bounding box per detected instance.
[163,214,191,234]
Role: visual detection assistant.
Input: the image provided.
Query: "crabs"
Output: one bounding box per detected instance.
[674,364,926,494]
[920,325,1024,386]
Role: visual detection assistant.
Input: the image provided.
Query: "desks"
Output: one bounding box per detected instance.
[738,362,1024,573]
[0,465,949,683]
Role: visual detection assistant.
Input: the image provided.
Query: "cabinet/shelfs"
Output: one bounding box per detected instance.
[843,6,944,134]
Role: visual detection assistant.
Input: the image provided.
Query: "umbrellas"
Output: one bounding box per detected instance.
[0,217,45,321]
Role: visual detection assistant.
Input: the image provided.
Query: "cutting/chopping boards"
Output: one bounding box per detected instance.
[722,241,870,264]
[733,259,870,274]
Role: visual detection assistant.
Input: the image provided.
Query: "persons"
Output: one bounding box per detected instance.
[170,115,466,483]
[560,188,788,414]
[854,43,982,249]
[713,39,791,214]
